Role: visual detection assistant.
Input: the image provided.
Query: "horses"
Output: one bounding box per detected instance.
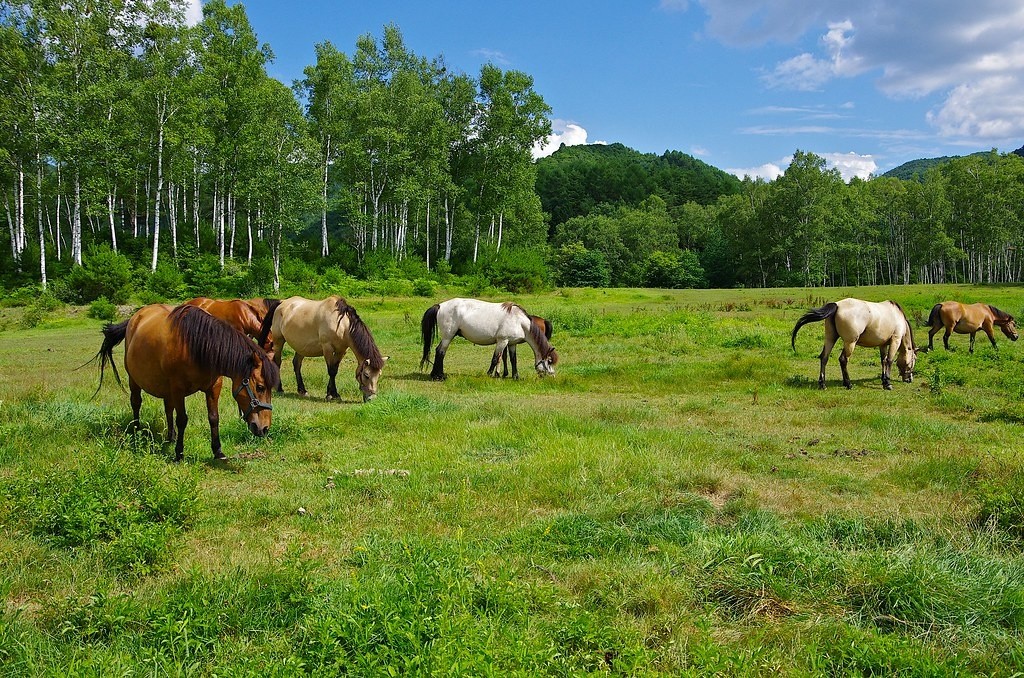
[69,294,389,465]
[788,298,917,392]
[419,297,560,383]
[922,301,1019,354]
